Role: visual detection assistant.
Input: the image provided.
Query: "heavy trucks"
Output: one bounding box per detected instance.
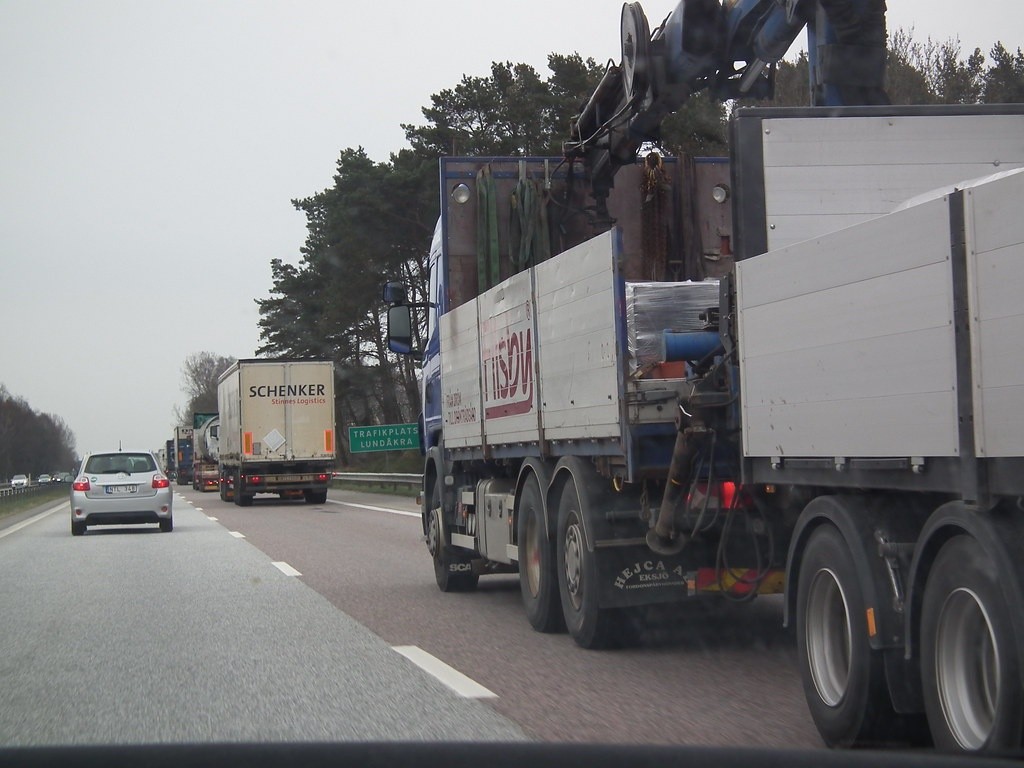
[379,1,1023,765]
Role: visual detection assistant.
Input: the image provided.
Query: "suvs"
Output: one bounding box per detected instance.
[38,474,51,486]
[10,475,29,490]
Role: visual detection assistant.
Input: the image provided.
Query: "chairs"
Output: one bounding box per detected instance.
[96,460,147,473]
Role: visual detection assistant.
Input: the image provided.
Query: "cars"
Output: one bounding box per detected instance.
[53,473,71,483]
[65,449,176,536]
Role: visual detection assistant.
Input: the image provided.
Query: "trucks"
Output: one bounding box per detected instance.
[171,425,194,485]
[158,440,175,482]
[187,412,220,491]
[210,358,337,507]
